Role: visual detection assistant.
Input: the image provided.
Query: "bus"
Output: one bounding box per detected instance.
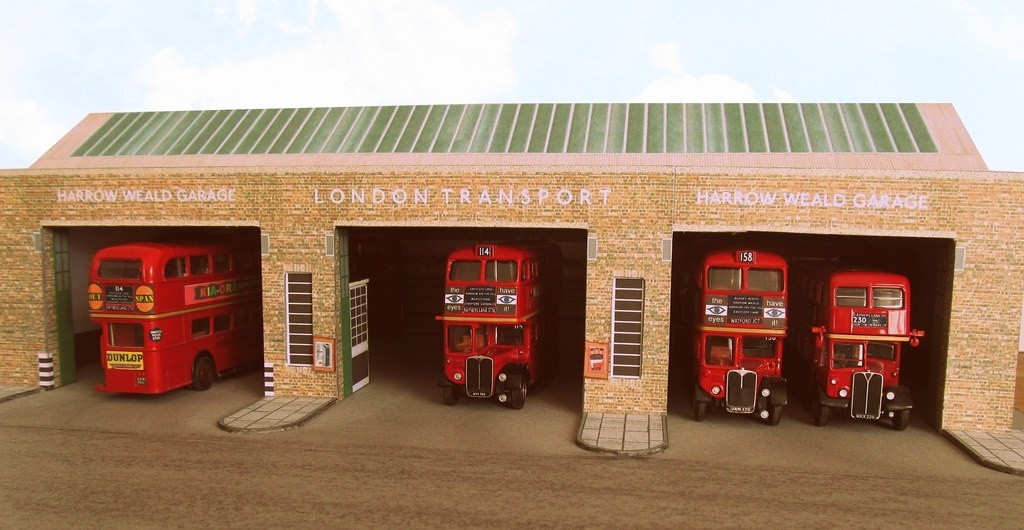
[794,272,926,431]
[435,243,545,410]
[688,248,790,427]
[87,242,265,397]
